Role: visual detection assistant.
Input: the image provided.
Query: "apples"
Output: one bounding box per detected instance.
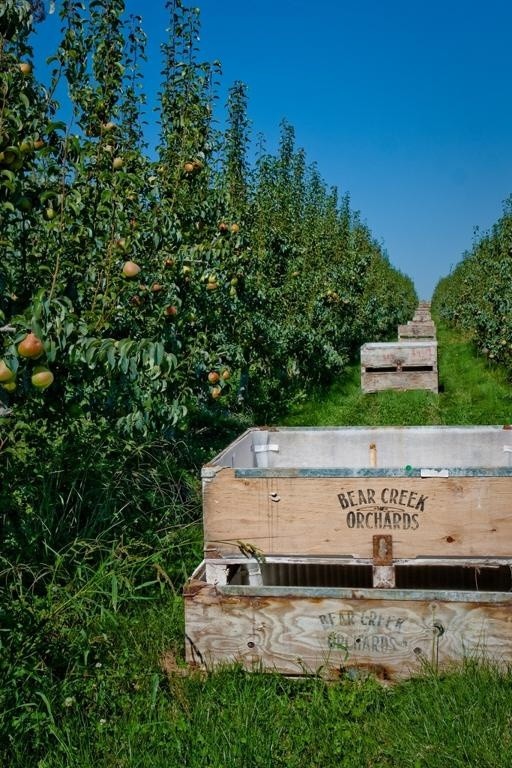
[0,55,298,400]
